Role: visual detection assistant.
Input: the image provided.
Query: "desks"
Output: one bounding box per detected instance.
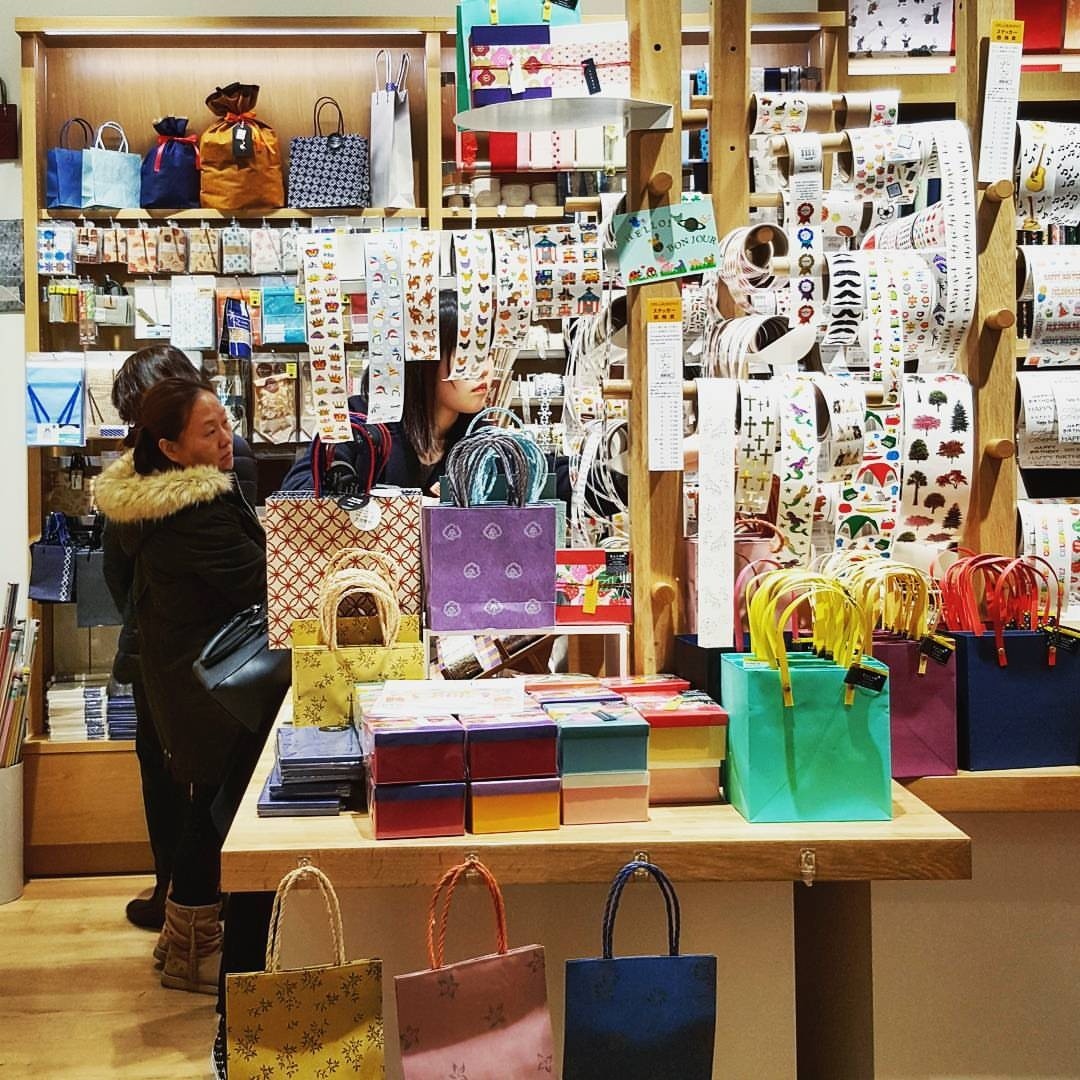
[219,685,971,1080]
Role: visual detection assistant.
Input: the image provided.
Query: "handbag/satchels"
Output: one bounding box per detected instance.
[225,865,385,1080]
[81,121,141,209]
[721,560,894,824]
[27,515,79,603]
[76,512,123,627]
[370,49,414,208]
[45,118,93,209]
[420,408,566,629]
[807,551,957,778]
[292,548,425,728]
[563,863,717,1080]
[393,860,555,1080]
[0,77,19,161]
[194,607,293,726]
[931,548,1080,771]
[685,519,785,634]
[287,97,369,209]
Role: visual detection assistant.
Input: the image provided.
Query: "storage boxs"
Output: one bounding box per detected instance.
[257,672,729,840]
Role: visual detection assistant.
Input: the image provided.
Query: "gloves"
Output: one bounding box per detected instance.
[265,410,422,652]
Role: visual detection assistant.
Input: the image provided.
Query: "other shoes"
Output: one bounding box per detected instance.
[127,886,167,932]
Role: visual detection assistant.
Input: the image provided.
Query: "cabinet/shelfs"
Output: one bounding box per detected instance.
[0,0,1080,879]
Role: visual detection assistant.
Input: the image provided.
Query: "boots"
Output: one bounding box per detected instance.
[153,921,169,961]
[162,890,223,995]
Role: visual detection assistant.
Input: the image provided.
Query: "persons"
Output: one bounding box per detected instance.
[92,344,268,995]
[281,289,628,526]
